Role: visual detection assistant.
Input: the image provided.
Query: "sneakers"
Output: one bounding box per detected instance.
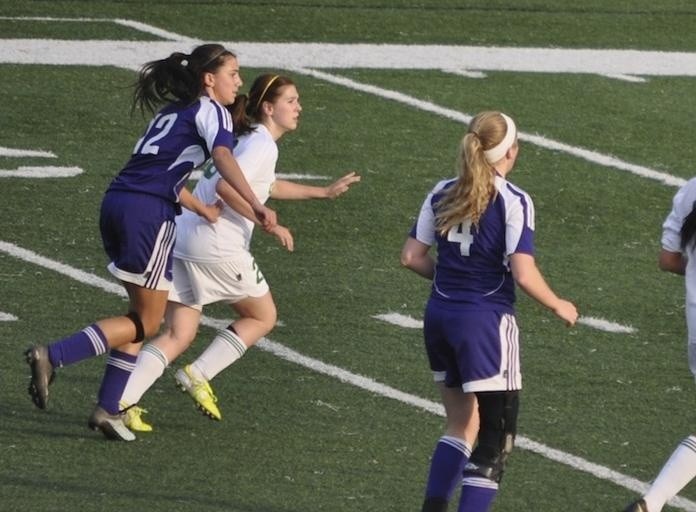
[87,404,136,441]
[23,345,55,410]
[173,363,221,421]
[117,401,152,432]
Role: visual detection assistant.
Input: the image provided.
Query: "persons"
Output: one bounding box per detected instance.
[618,178,696,512]
[119,74,362,434]
[399,109,579,511]
[22,44,278,442]
